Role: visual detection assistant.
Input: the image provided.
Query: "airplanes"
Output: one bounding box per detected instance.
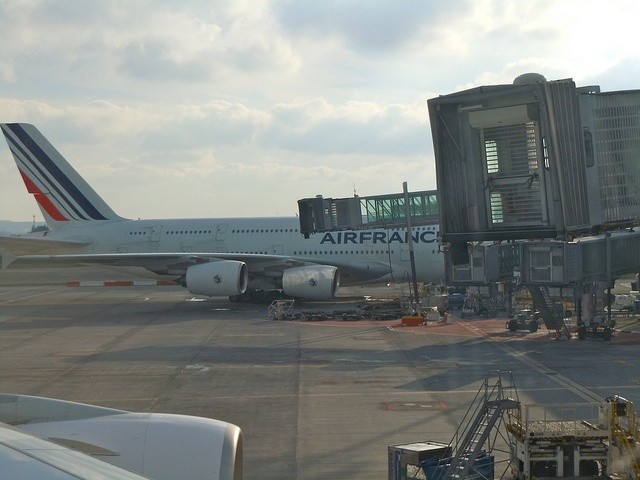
[1,393,242,479]
[1,124,444,299]
